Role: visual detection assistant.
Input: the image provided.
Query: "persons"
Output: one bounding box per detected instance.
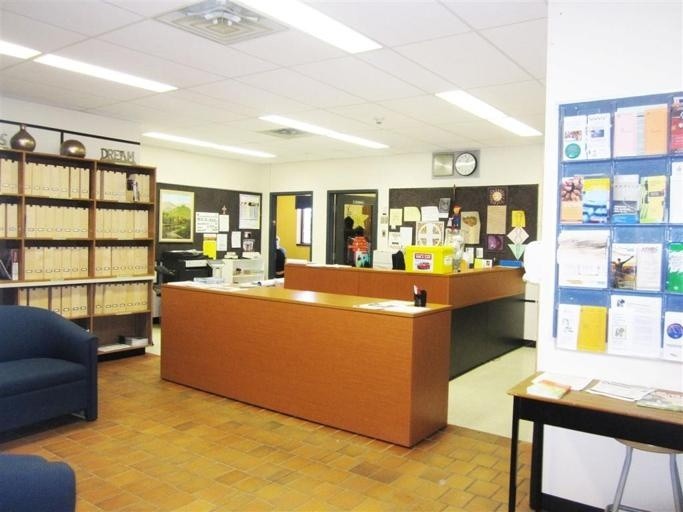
[276,235,286,279]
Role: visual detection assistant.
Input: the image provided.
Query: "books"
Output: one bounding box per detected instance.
[558,227,609,289]
[609,241,663,291]
[560,113,610,160]
[555,301,606,353]
[240,277,283,287]
[662,309,683,357]
[666,241,682,294]
[525,368,682,410]
[667,96,683,158]
[613,103,671,157]
[669,161,683,224]
[560,172,609,224]
[608,293,661,358]
[611,174,666,223]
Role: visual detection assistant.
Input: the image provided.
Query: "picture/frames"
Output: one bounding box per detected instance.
[159,190,195,243]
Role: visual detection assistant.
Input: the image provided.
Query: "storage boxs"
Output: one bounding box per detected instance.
[403,245,456,274]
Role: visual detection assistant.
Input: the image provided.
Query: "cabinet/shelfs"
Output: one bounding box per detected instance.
[160,282,452,447]
[2,149,156,356]
[449,268,525,310]
[285,263,449,305]
[553,91,682,362]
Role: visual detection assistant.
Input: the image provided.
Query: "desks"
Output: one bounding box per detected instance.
[507,371,683,512]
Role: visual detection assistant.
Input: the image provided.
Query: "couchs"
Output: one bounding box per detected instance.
[1,454,75,511]
[0,306,99,432]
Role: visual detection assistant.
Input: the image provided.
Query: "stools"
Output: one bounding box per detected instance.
[606,439,683,510]
